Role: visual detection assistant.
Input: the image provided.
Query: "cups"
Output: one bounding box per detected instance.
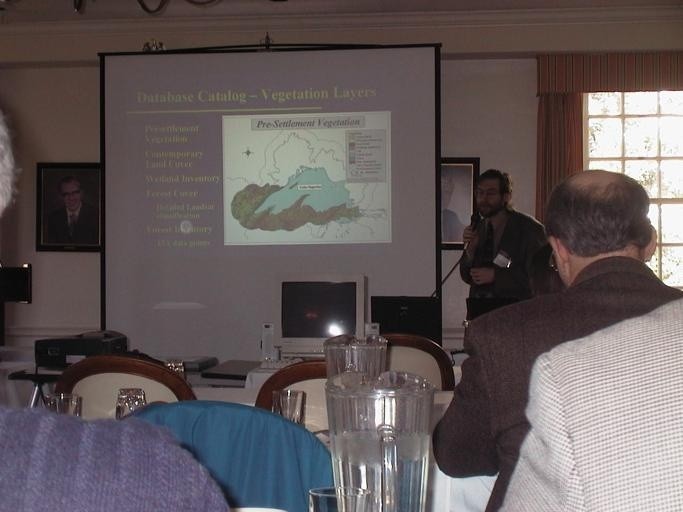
[326,373,435,512]
[307,488,364,512]
[269,389,306,425]
[45,394,78,415]
[324,335,388,373]
[116,390,145,420]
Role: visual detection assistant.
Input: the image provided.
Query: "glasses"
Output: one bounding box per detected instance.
[61,191,80,197]
[549,252,559,272]
[473,189,501,198]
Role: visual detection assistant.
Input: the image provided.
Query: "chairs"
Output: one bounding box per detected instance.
[121,398,333,512]
[326,334,455,389]
[59,358,198,422]
[257,357,327,433]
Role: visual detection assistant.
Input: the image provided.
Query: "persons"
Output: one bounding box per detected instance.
[1,114,231,512]
[499,295,683,512]
[460,168,548,314]
[43,178,99,245]
[441,175,465,242]
[433,170,683,512]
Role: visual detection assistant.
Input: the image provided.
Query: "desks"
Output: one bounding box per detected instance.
[9,365,454,428]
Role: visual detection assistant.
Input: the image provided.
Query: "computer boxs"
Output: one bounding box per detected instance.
[370,296,442,346]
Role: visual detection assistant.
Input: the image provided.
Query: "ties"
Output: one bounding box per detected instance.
[479,222,494,297]
[70,212,77,233]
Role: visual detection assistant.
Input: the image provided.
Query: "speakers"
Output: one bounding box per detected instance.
[0,263,32,305]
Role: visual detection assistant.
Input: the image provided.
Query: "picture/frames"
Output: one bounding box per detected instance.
[437,157,480,251]
[35,159,102,253]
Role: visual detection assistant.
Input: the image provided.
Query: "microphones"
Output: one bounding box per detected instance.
[464,213,485,250]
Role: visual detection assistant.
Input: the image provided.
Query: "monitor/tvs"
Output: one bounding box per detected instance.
[274,272,364,361]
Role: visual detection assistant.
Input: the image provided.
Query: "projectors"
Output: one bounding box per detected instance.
[35,330,127,371]
